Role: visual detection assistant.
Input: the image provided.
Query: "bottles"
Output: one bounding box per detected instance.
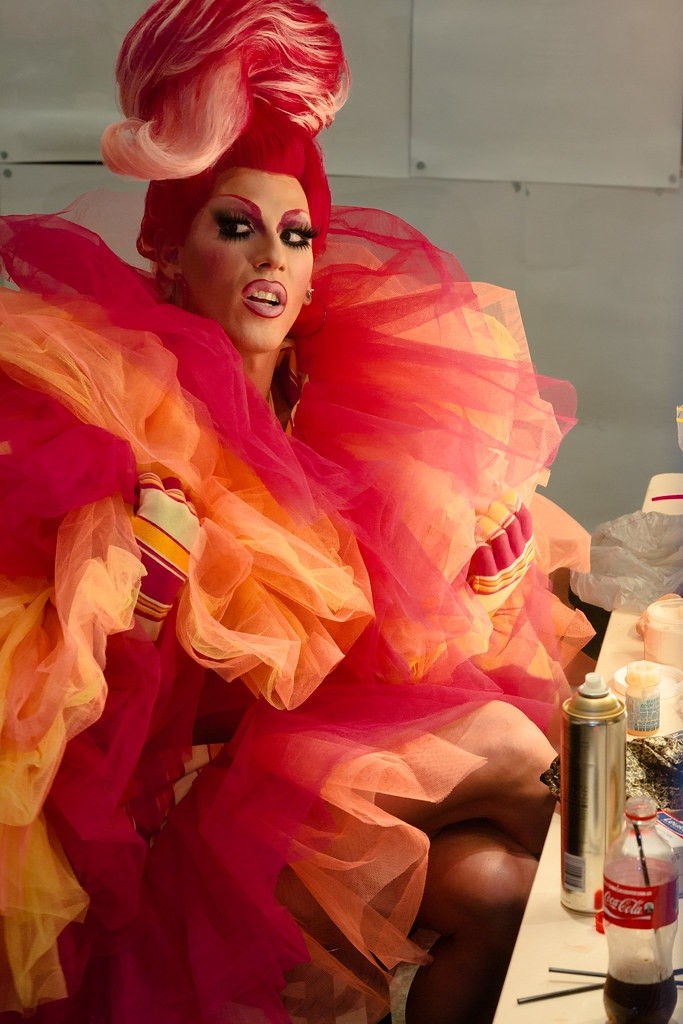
[559,674,626,918]
[601,796,679,1024]
[628,660,661,736]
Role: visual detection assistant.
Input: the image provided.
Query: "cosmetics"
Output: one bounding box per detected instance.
[625,662,661,737]
[644,599,683,671]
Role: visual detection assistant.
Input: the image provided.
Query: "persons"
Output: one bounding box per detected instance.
[0,0,598,1024]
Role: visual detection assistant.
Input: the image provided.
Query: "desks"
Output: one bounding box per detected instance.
[489,470,683,1024]
